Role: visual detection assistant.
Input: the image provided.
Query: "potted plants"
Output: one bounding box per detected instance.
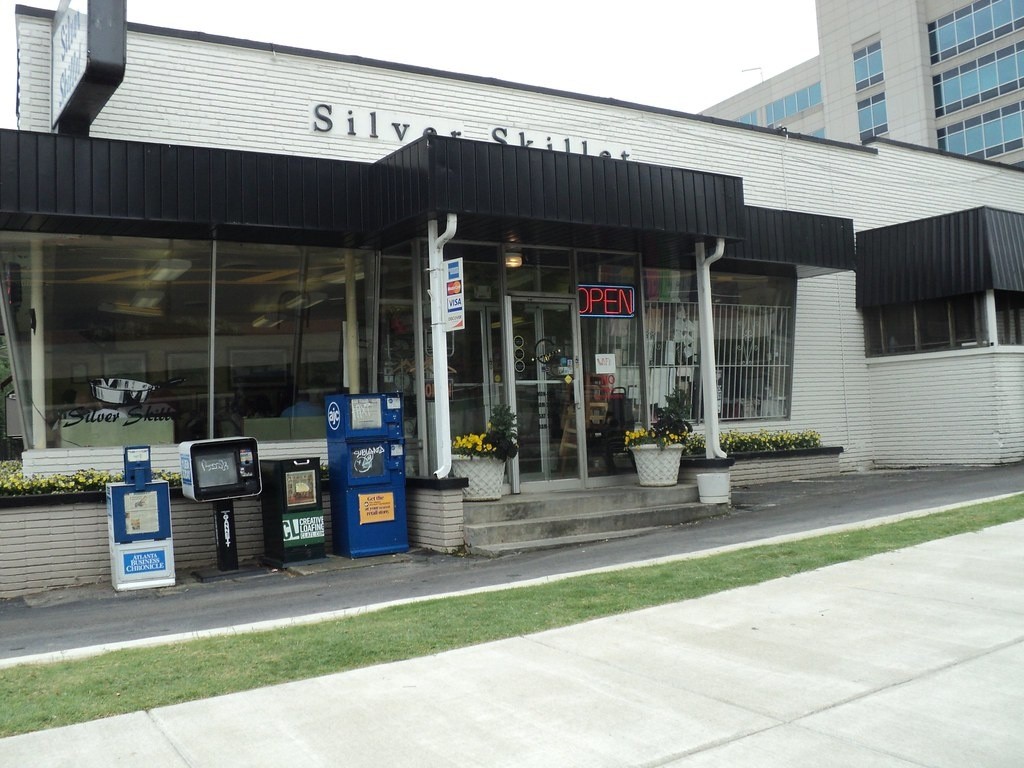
[629,386,693,487]
[452,403,521,501]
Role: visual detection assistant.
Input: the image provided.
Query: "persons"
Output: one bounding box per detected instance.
[46,390,87,428]
[282,375,331,416]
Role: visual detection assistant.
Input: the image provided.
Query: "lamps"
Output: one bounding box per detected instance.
[502,231,523,267]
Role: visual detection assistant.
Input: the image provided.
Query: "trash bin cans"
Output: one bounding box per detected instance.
[697,472,730,504]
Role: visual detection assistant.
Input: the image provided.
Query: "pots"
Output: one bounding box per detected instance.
[89,376,189,406]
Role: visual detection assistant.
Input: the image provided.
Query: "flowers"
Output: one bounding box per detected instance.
[451,422,497,461]
[623,428,688,446]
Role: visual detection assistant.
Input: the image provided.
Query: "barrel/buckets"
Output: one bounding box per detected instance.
[696,472,730,504]
